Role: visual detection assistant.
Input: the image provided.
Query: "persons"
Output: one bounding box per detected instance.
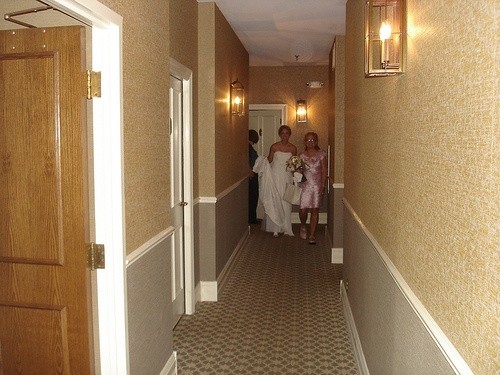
[253,125,327,245]
[248,129,261,225]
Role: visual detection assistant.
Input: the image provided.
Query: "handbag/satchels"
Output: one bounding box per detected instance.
[283,183,303,206]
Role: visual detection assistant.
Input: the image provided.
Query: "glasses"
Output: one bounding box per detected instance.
[307,138,313,142]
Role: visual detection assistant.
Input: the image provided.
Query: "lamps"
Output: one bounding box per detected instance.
[306,80,324,88]
[230,80,245,116]
[364,0,405,77]
[296,100,306,122]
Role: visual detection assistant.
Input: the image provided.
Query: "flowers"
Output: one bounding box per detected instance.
[286,156,311,171]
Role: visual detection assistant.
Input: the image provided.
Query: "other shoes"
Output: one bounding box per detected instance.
[300,227,307,239]
[273,232,278,238]
[308,236,316,244]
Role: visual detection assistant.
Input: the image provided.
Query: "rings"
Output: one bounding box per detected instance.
[321,192,324,194]
